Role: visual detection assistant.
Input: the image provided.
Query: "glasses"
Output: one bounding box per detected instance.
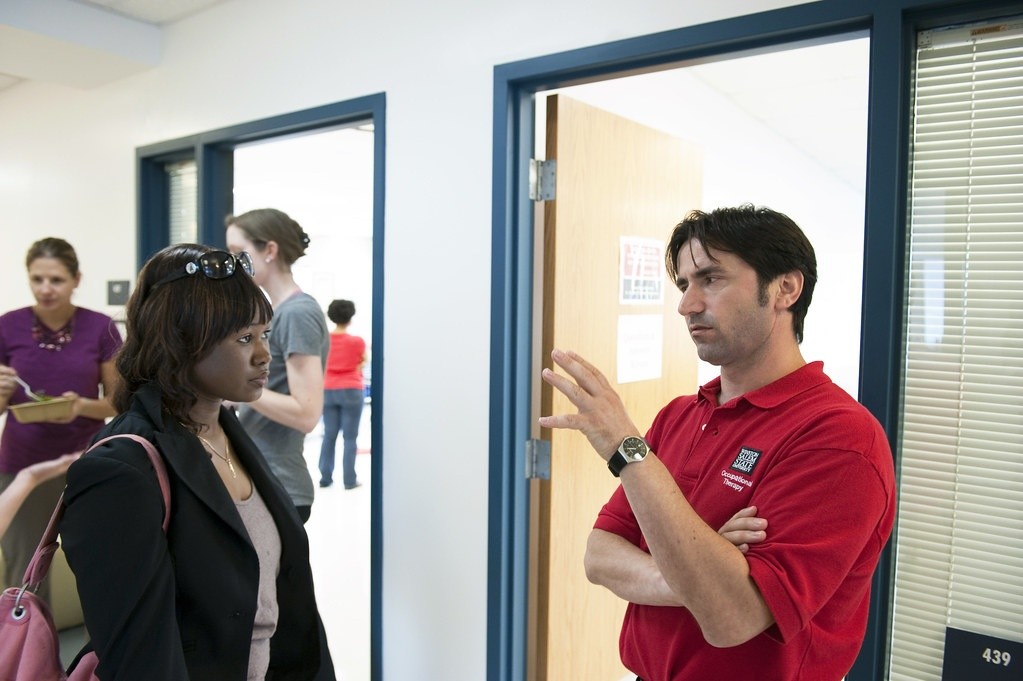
[148,250,255,294]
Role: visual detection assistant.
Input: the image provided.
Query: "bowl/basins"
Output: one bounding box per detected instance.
[6,396,79,424]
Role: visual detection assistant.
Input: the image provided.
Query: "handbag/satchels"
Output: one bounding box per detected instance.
[0,434,172,681]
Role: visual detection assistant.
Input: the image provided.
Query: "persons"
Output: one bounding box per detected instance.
[538,205,896,681]
[318,300,366,489]
[57,243,336,681]
[0,451,83,538]
[0,237,123,588]
[222,208,330,525]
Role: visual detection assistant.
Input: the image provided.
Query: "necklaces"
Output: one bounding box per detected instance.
[163,403,236,479]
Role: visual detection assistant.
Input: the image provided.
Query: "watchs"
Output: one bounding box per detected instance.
[608,436,652,477]
